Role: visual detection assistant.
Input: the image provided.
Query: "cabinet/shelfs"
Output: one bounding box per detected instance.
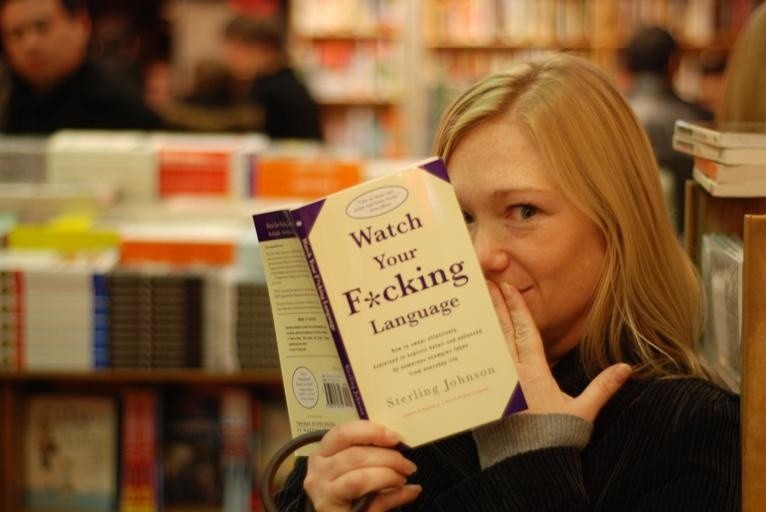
[1,368,294,512]
[670,0,765,512]
